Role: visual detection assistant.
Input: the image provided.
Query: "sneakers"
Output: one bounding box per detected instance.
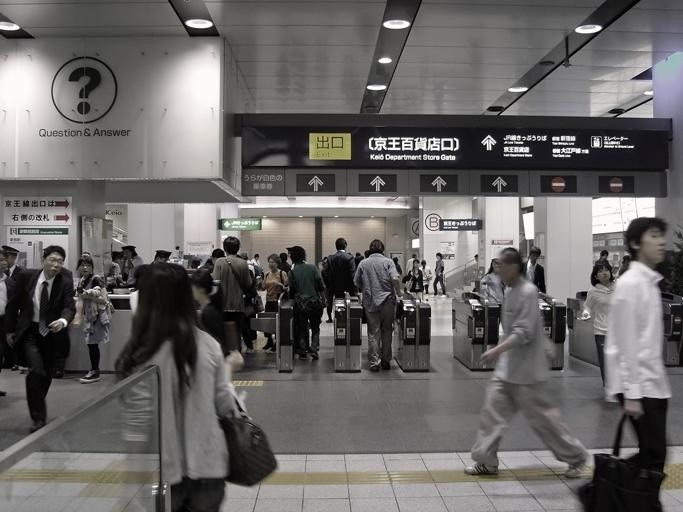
[371,365,380,373]
[381,360,390,370]
[246,347,254,354]
[299,354,309,359]
[310,351,319,359]
[463,462,499,475]
[80,368,100,382]
[564,450,591,479]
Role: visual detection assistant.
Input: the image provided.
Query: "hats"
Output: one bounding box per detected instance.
[286,246,305,260]
[1,245,20,254]
[156,249,172,261]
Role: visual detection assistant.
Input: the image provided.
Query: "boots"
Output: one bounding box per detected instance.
[263,338,276,351]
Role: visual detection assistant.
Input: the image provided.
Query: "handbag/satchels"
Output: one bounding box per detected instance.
[406,280,414,290]
[294,290,328,314]
[219,417,277,487]
[243,283,257,306]
[578,454,667,512]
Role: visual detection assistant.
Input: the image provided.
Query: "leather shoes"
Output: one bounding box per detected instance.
[29,419,44,433]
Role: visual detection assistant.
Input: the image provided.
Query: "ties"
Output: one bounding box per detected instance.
[39,281,50,337]
[7,267,11,276]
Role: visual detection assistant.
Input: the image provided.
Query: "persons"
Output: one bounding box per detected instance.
[603,217,672,472]
[113,262,246,511]
[430,252,447,298]
[464,245,591,478]
[596,250,611,265]
[524,246,547,295]
[471,253,479,293]
[0,235,432,384]
[582,260,623,403]
[479,257,505,350]
[4,246,76,434]
